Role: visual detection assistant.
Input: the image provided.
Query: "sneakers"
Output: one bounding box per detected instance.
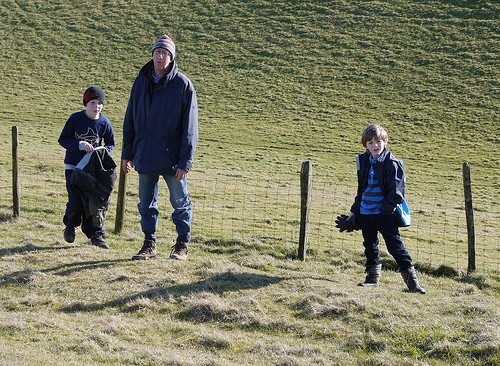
[132,239,157,260]
[170,241,189,260]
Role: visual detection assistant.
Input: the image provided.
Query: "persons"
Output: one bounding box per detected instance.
[121,34,198,260]
[346,124,427,294]
[57,87,118,248]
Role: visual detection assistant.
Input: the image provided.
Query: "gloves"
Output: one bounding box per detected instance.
[334,213,360,233]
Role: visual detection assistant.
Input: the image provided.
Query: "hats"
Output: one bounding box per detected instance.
[151,34,176,61]
[83,86,105,105]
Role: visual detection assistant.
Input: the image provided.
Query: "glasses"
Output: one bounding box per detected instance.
[152,49,172,56]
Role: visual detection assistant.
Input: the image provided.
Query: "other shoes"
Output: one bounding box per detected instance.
[357,280,381,287]
[408,286,427,294]
[63,225,76,243]
[91,235,111,249]
